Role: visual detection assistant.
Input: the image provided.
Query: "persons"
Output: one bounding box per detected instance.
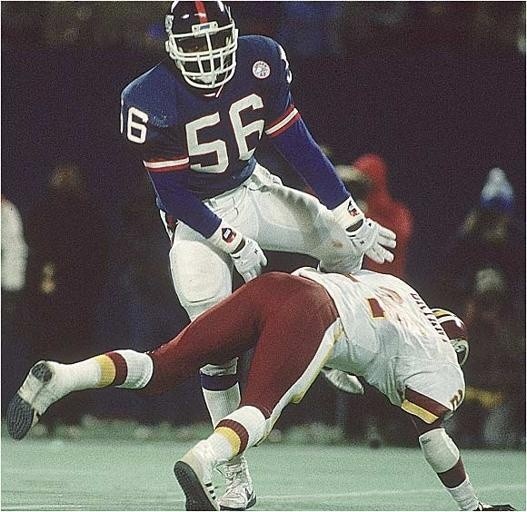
[1,119,526,450]
[2,267,519,512]
[115,2,398,512]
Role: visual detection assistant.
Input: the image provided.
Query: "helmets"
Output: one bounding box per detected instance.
[431,307,470,366]
[165,1,238,46]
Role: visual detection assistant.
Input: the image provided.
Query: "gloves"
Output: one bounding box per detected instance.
[343,216,397,265]
[473,501,517,511]
[228,237,268,283]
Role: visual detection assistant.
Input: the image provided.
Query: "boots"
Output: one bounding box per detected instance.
[6,360,71,441]
[219,452,256,511]
[173,440,221,511]
